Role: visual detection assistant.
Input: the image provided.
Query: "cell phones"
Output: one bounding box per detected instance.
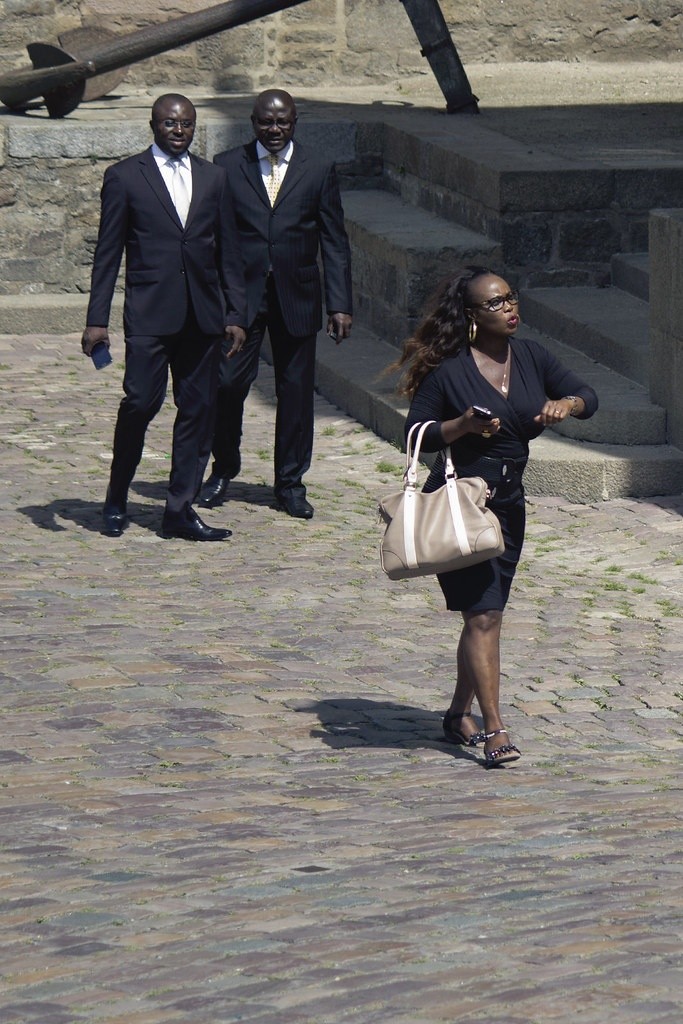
[91,342,113,370]
[472,406,492,421]
[330,326,337,340]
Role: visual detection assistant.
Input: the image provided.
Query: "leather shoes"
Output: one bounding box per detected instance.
[195,474,232,506]
[162,511,235,539]
[278,495,317,520]
[99,511,128,535]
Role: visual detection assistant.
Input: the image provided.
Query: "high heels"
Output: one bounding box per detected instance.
[441,707,486,747]
[480,727,521,767]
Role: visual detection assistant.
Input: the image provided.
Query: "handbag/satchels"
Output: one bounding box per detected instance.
[378,419,507,582]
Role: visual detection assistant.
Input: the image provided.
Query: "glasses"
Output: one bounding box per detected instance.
[152,117,196,131]
[253,116,297,131]
[472,288,522,313]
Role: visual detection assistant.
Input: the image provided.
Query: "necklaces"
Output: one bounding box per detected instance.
[501,360,507,392]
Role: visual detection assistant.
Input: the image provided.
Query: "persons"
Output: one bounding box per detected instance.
[393,266,599,766]
[82,93,246,539]
[198,89,354,520]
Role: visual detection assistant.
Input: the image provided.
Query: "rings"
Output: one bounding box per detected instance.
[482,430,491,438]
[555,411,560,413]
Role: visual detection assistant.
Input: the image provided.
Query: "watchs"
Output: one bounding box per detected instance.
[562,396,578,413]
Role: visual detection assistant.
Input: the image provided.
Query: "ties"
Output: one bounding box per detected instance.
[166,158,191,226]
[266,154,281,208]
